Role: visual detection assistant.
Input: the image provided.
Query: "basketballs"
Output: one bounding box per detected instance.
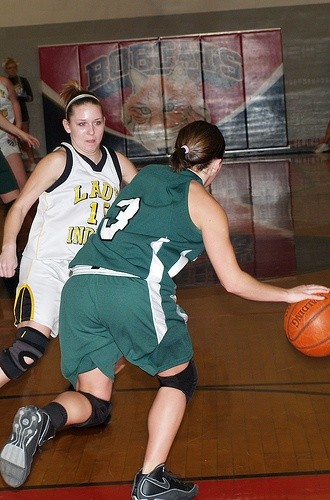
[281,291,329,361]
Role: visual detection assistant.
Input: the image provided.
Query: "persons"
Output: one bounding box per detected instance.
[0,55,41,218]
[0,120,330,500]
[0,78,138,389]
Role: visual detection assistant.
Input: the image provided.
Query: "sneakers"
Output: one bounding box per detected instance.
[131,463,199,500]
[0,405,55,488]
